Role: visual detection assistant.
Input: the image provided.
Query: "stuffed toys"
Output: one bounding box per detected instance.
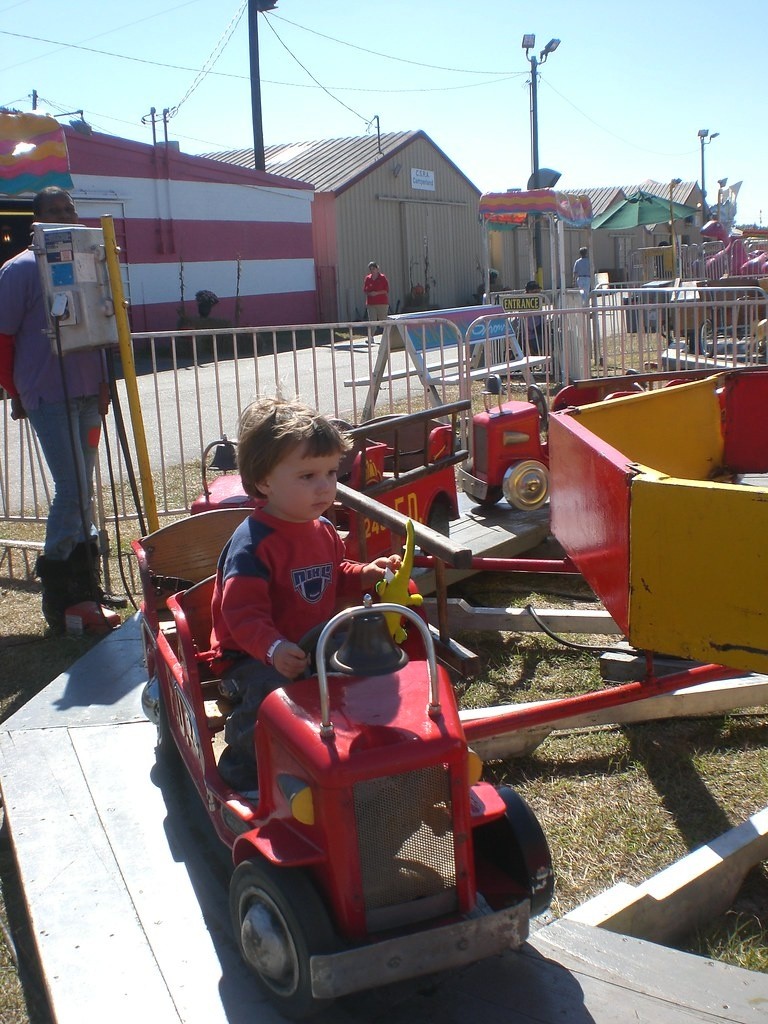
[376,518,422,644]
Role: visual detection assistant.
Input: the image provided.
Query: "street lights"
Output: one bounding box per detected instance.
[670,179,682,279]
[522,33,562,293]
[698,129,721,226]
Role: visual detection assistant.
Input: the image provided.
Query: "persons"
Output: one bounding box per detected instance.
[363,262,389,343]
[210,398,402,809]
[518,281,552,354]
[573,247,590,301]
[0,188,127,627]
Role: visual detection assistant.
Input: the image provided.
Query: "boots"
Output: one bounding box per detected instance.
[34,555,82,628]
[67,548,128,609]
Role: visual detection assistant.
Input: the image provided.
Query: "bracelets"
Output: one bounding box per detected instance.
[266,636,287,665]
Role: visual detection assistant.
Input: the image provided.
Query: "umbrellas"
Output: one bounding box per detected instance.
[588,188,702,231]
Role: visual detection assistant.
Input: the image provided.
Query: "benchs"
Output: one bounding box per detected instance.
[344,304,553,424]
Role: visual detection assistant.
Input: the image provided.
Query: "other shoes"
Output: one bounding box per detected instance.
[365,338,374,344]
